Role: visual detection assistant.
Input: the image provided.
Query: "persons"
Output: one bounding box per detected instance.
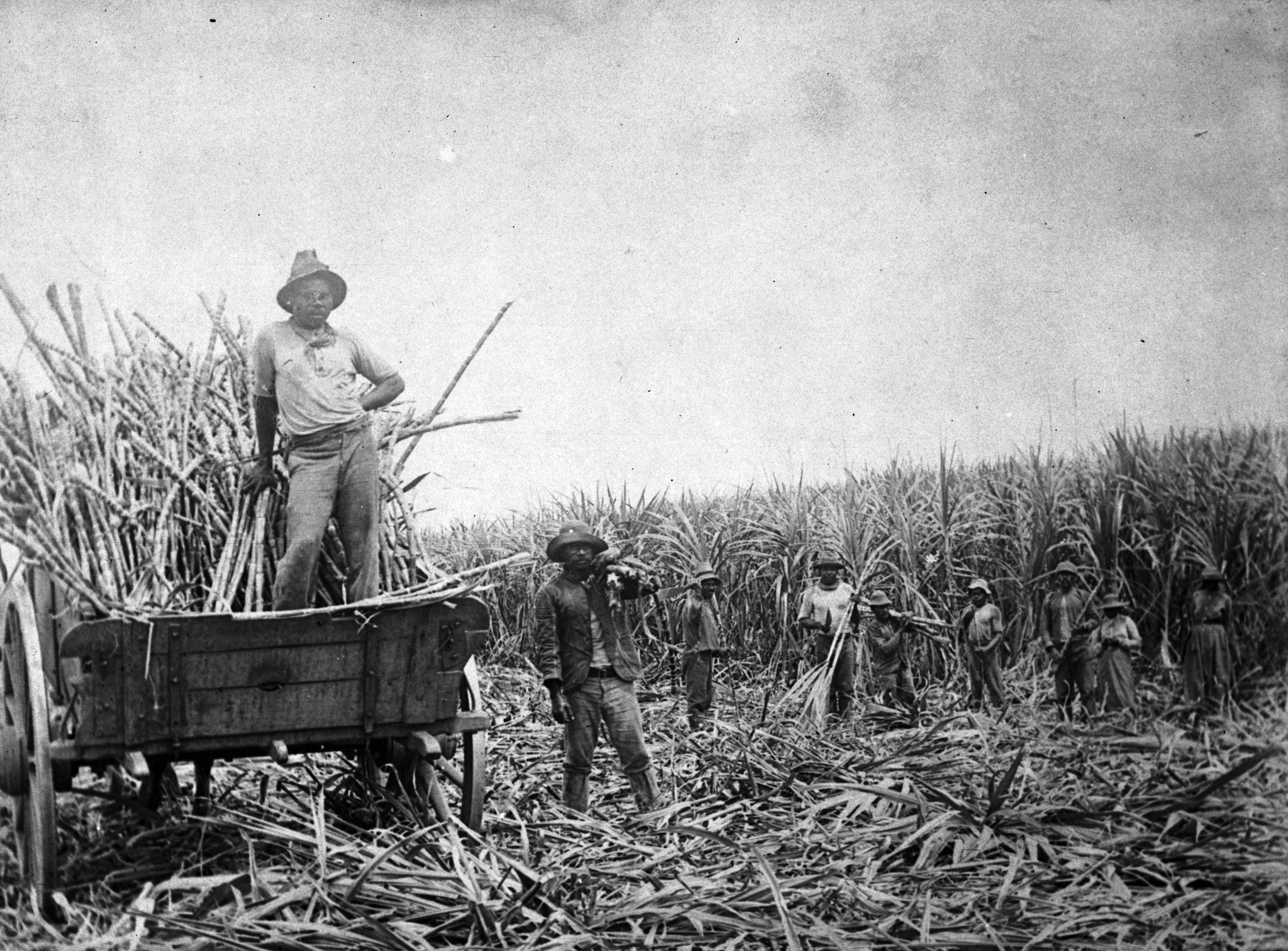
[234,250,407,611]
[1184,568,1244,702]
[533,523,665,820]
[1090,592,1148,715]
[955,578,1010,709]
[798,551,860,723]
[672,561,730,731]
[862,592,919,710]
[1042,559,1099,728]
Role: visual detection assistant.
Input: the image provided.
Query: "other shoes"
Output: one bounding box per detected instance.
[690,721,712,733]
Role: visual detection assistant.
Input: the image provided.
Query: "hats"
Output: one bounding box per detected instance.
[813,551,845,570]
[546,520,609,562]
[276,250,348,313]
[1192,565,1224,583]
[1054,560,1079,577]
[689,562,723,590]
[1096,593,1130,609]
[868,590,893,606]
[967,578,991,595]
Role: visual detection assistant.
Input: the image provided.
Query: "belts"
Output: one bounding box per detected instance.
[1193,617,1222,624]
